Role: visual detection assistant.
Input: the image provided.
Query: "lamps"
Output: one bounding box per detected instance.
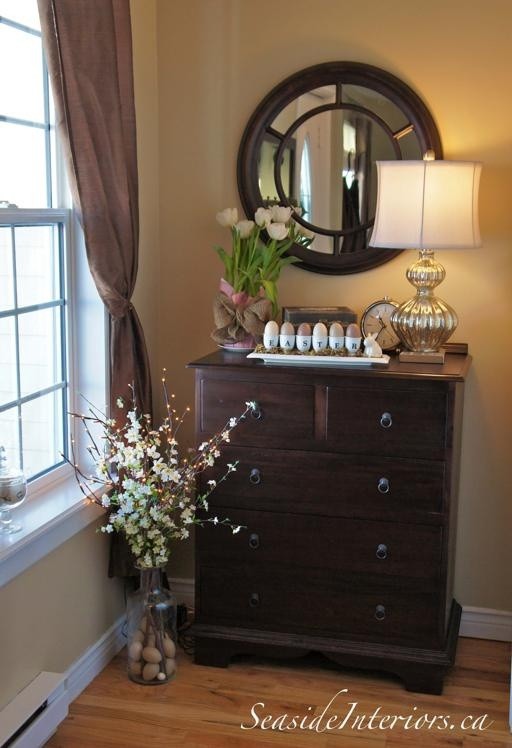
[368,147,486,364]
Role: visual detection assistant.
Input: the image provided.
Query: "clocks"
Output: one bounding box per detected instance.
[361,295,404,355]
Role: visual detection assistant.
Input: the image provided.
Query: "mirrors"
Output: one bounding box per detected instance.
[237,58,445,274]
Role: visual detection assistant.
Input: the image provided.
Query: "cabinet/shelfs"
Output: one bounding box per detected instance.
[193,372,451,649]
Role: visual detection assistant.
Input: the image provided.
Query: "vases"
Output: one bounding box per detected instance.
[122,554,184,686]
[211,292,272,353]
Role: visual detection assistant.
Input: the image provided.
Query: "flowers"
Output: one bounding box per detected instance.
[212,203,304,305]
[291,205,317,247]
[56,376,255,673]
